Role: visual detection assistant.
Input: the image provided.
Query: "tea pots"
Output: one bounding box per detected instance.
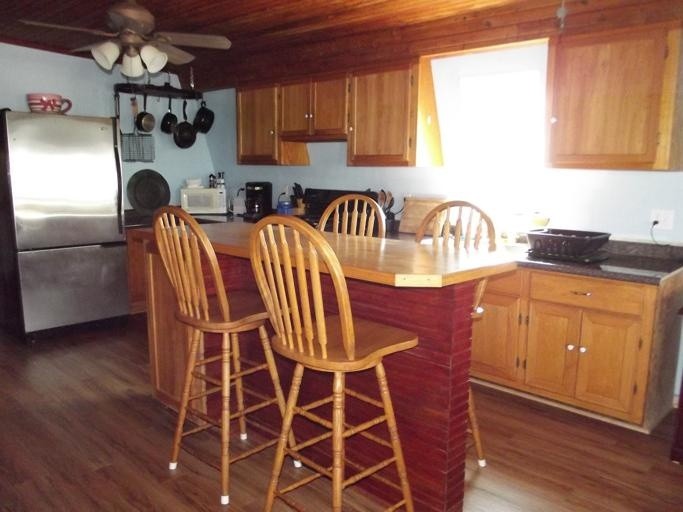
[276,192,291,218]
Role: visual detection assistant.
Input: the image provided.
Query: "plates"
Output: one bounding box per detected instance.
[185,185,205,188]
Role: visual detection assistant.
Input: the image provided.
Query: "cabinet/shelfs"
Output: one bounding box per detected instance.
[519,266,683,426]
[545,21,683,172]
[470,265,517,389]
[236,80,311,167]
[347,57,435,168]
[278,70,347,142]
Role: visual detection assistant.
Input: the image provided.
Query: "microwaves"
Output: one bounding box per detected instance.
[179,185,230,216]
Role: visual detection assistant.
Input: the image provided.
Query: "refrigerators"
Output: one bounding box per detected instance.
[0,109,134,341]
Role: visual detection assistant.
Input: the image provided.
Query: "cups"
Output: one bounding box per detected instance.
[185,177,202,186]
[25,90,73,118]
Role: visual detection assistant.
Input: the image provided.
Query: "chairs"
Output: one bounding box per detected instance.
[150,205,303,505]
[316,194,386,239]
[415,201,498,468]
[248,213,419,511]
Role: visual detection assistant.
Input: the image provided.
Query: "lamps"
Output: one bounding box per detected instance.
[91,32,169,78]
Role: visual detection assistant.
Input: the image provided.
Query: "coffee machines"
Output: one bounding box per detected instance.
[242,181,273,224]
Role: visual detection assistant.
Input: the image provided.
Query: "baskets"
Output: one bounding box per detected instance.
[526,227,611,260]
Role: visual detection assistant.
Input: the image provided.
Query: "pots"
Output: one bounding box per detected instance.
[133,89,215,150]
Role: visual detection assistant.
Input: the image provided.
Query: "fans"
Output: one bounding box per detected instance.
[15,0,232,66]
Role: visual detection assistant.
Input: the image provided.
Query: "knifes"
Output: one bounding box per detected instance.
[290,182,303,207]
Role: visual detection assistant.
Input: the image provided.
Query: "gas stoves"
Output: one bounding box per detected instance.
[298,209,367,233]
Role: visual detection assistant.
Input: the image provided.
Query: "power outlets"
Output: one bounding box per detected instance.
[403,194,413,205]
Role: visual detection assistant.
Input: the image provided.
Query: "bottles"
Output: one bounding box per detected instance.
[217,171,225,189]
[208,172,217,187]
